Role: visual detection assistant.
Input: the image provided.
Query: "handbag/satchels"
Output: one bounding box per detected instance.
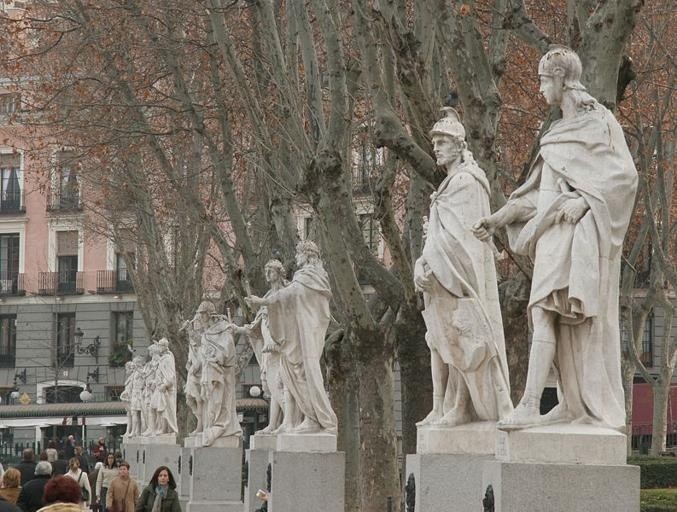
[82,487,88,501]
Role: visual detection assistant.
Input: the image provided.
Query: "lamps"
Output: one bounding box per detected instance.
[79,368,98,401]
[10,367,26,400]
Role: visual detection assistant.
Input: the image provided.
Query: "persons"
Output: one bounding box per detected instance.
[184,240,338,447]
[1,435,181,512]
[415,108,513,427]
[472,45,639,431]
[120,338,177,434]
